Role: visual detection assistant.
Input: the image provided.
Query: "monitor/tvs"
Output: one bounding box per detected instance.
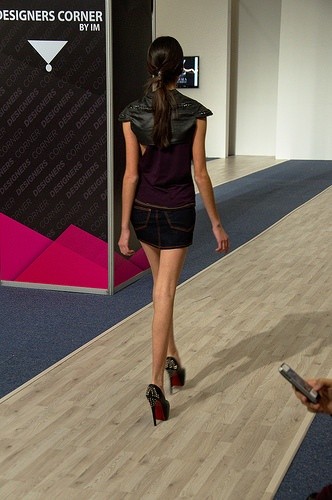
[175,56,199,88]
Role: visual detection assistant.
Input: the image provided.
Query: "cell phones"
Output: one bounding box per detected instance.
[279,363,320,404]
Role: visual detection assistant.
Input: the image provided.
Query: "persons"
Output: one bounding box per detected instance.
[117,37,229,427]
[293,378,332,415]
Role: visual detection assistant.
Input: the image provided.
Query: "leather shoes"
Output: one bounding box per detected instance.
[165,356,186,394]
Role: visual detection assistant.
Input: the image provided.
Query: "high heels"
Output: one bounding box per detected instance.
[146,383,170,425]
